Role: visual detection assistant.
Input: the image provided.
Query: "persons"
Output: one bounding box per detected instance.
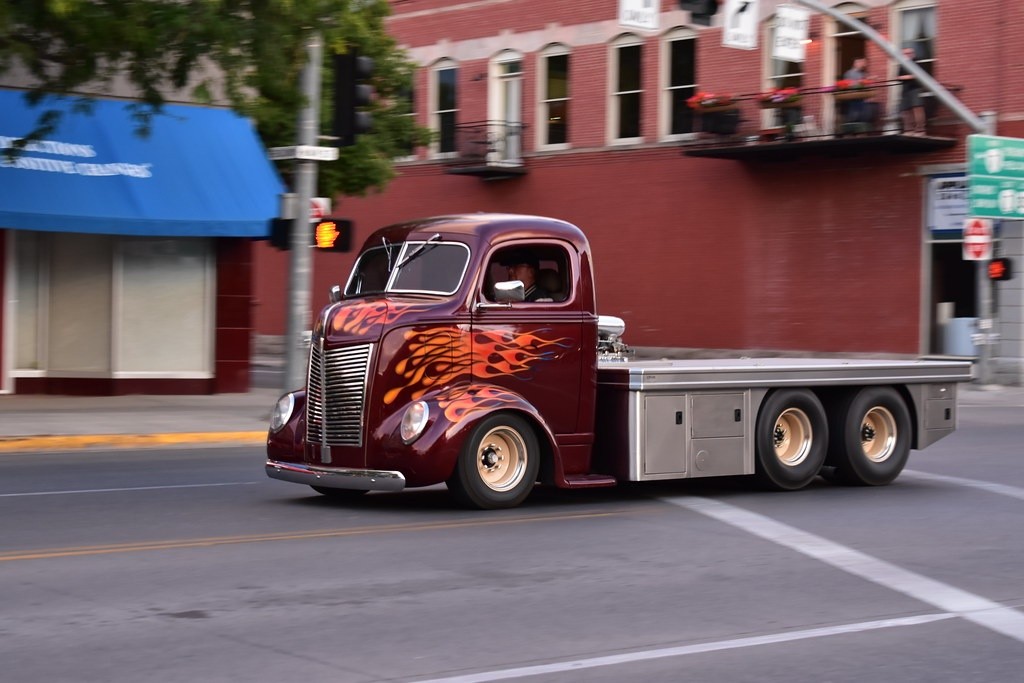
[481,253,555,303]
[893,47,928,136]
[842,54,877,132]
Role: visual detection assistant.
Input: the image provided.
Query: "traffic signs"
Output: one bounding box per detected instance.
[965,129,1023,223]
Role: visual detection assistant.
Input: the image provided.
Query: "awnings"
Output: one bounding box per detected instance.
[0,85,289,237]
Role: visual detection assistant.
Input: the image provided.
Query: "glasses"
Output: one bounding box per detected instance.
[505,263,534,270]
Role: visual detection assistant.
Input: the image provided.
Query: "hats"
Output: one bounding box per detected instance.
[499,251,539,266]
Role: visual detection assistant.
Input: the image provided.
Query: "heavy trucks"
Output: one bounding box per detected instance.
[266,211,984,510]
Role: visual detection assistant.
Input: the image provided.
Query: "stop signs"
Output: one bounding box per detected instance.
[961,214,993,257]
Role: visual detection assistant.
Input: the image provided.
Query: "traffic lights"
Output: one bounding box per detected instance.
[311,222,350,253]
[336,49,379,144]
[983,256,1012,284]
